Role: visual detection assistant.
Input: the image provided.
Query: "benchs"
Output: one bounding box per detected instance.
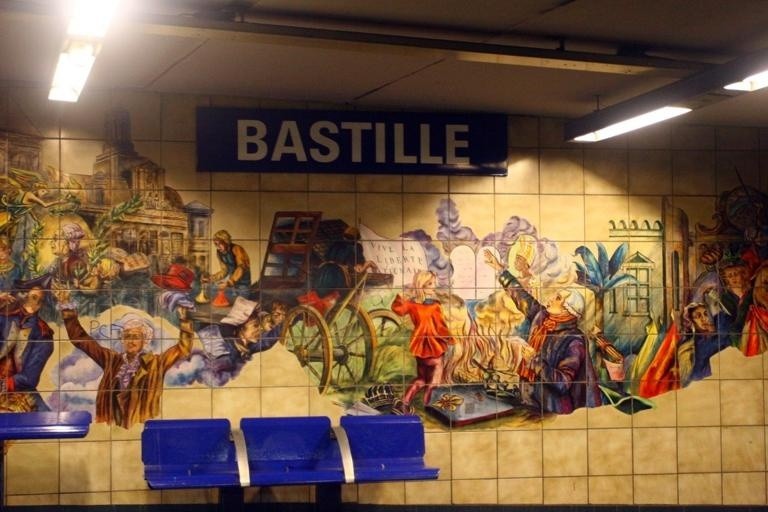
[141,413,439,504]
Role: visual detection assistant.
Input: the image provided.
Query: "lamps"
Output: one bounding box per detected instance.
[558,47,768,152]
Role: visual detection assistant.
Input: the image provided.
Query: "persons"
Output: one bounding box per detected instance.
[311,226,378,302]
[475,236,539,327]
[0,180,70,274]
[389,270,456,418]
[239,300,295,346]
[47,276,195,432]
[482,246,607,417]
[204,228,252,291]
[704,256,757,350]
[45,223,87,285]
[669,298,732,388]
[0,284,55,413]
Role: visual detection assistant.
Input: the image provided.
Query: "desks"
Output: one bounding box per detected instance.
[0,412,92,506]
